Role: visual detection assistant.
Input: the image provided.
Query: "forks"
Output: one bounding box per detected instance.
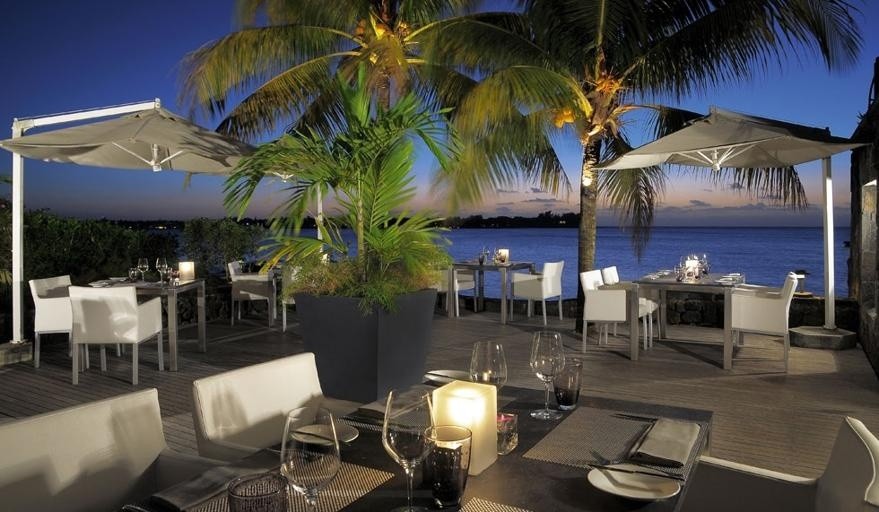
[588,450,684,477]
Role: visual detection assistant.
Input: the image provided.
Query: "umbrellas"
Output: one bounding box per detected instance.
[575,105,876,330]
[0,97,288,344]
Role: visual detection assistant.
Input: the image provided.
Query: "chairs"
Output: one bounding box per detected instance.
[676,414,877,509]
[511,260,564,327]
[579,264,799,372]
[424,261,477,314]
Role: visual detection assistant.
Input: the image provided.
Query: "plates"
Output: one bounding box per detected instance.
[586,462,682,501]
[290,423,360,447]
[713,273,741,287]
[423,369,470,385]
[87,276,128,288]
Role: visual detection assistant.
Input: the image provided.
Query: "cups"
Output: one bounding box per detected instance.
[422,425,472,512]
[497,414,519,456]
[477,246,505,266]
[674,252,709,283]
[469,340,508,391]
[227,472,289,512]
[551,356,584,411]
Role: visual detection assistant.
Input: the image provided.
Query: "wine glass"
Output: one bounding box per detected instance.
[527,330,566,421]
[279,406,340,512]
[382,388,436,512]
[129,256,180,287]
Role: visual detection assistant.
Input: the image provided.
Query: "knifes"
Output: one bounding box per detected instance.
[615,413,658,422]
[425,372,467,382]
[292,431,352,447]
[587,463,684,482]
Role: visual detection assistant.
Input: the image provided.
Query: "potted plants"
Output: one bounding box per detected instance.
[281,261,445,407]
